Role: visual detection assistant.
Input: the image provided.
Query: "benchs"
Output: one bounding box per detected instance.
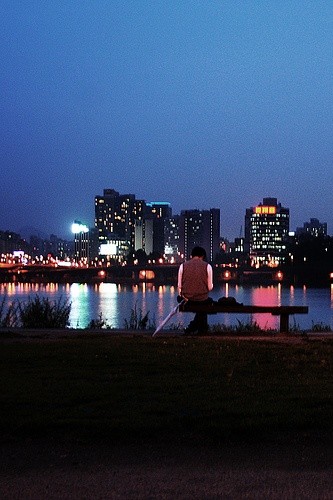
[180,294,310,331]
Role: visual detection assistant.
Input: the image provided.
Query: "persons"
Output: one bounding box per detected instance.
[175,243,214,331]
